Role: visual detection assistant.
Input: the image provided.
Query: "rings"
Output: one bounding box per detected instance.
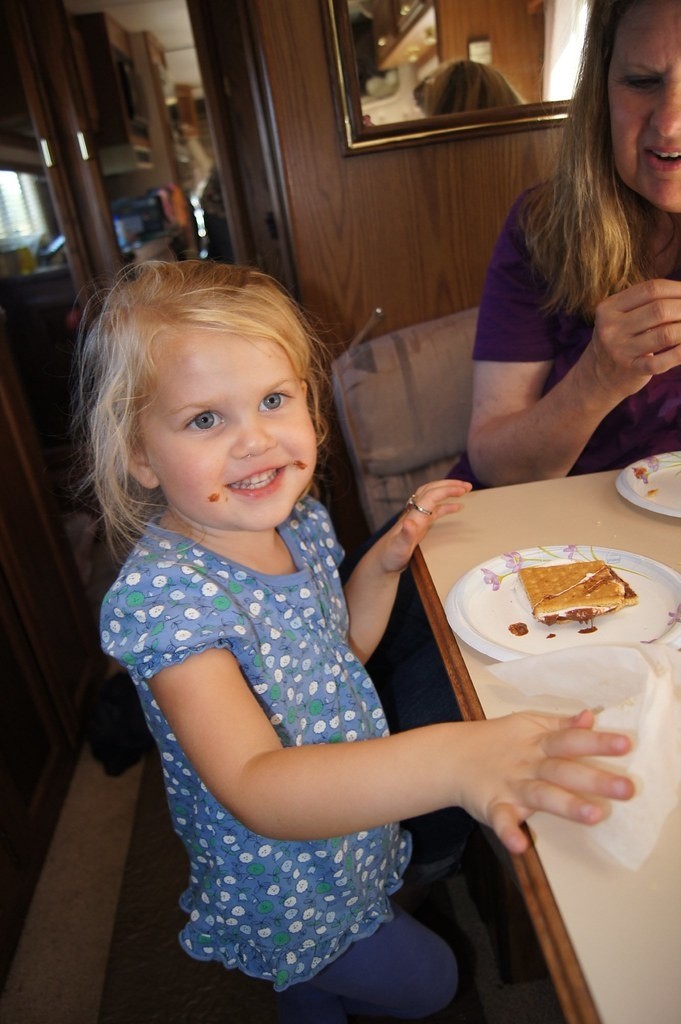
[406,495,433,516]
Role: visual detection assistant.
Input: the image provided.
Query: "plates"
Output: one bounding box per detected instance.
[615,450,681,519]
[444,545,681,662]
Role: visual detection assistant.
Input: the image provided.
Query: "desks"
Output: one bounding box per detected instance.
[404,468,681,1024]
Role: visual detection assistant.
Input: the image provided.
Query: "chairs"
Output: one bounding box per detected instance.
[331,303,484,532]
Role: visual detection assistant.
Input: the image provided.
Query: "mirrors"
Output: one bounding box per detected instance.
[319,0,596,159]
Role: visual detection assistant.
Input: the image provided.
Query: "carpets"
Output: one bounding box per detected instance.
[95,737,567,1024]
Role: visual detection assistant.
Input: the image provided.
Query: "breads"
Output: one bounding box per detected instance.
[518,561,639,626]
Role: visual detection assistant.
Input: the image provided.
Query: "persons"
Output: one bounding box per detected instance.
[78,261,635,1024]
[422,60,524,117]
[338,0,681,884]
